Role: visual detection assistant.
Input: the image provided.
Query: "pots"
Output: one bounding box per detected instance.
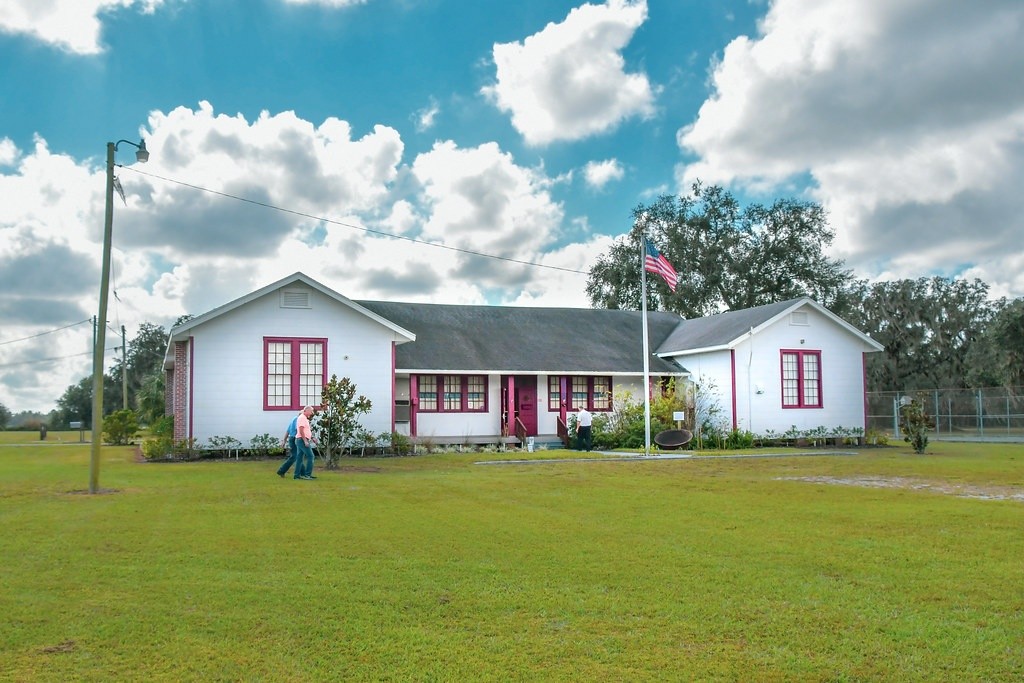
[654,430,694,449]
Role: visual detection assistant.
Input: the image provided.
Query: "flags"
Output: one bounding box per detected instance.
[644,239,677,293]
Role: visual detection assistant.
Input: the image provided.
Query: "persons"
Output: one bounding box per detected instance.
[576,406,592,452]
[277,406,318,479]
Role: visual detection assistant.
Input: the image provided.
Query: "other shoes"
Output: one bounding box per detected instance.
[300,474,305,476]
[277,469,285,478]
[305,474,317,479]
[294,476,304,480]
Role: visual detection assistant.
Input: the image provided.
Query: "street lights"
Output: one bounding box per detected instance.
[90,139,150,494]
